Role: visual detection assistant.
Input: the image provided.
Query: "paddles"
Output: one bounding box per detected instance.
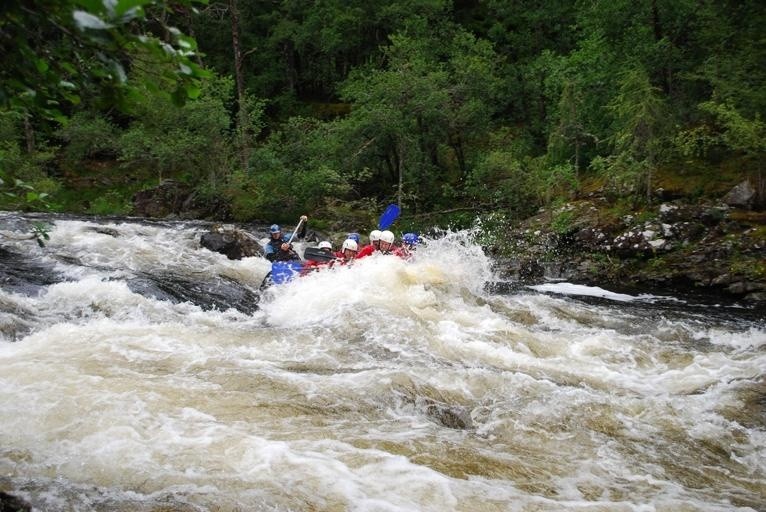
[259,218,304,291]
[272,247,350,285]
[379,204,400,232]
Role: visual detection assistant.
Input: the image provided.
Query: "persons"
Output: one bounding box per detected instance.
[263,213,308,263]
[299,230,422,275]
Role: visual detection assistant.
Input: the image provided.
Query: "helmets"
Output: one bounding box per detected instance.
[347,233,359,242]
[369,230,381,246]
[270,224,280,233]
[342,239,358,253]
[318,241,332,249]
[402,233,418,244]
[380,230,394,250]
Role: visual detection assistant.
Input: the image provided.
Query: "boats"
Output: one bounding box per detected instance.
[272,260,450,284]
